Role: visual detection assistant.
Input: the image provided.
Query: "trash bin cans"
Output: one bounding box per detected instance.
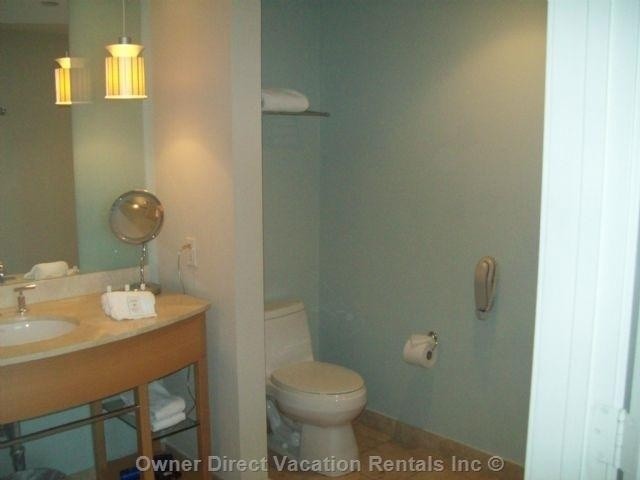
[4,467,69,480]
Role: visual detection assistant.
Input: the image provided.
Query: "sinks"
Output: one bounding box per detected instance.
[0,314,83,349]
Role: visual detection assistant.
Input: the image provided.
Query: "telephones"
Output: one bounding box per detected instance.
[475,257,495,312]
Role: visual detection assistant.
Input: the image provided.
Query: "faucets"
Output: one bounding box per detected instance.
[0,263,18,284]
[14,284,38,316]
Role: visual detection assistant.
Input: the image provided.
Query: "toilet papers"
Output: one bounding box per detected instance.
[403,332,436,368]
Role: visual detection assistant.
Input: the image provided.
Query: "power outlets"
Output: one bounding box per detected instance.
[185,238,197,268]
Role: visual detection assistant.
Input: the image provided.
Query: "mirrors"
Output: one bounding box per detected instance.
[0,0,161,309]
[110,190,161,295]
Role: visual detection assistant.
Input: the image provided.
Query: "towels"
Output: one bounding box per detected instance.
[120,380,185,420]
[102,291,157,320]
[124,405,185,433]
[23,261,69,280]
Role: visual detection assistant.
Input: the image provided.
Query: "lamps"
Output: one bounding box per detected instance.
[54,50,94,105]
[104,0,148,99]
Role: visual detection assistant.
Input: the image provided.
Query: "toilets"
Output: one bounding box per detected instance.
[265,299,367,477]
